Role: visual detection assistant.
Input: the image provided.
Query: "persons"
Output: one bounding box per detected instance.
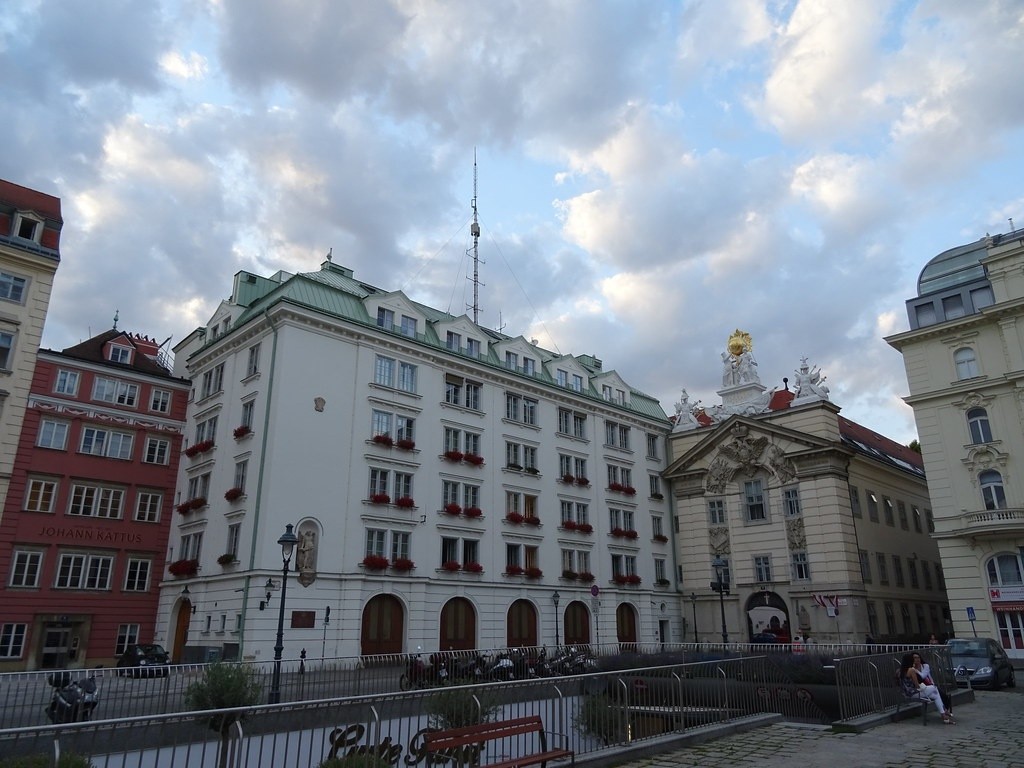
[678,388,690,424]
[299,530,314,569]
[738,347,756,383]
[720,350,735,386]
[897,652,957,726]
[865,634,874,655]
[795,356,817,397]
[929,634,938,644]
[798,605,810,628]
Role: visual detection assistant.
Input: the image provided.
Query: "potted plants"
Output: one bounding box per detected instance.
[506,462,540,474]
[217,553,238,566]
[648,491,670,587]
[561,470,590,485]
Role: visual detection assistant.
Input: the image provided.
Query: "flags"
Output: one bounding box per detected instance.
[813,594,838,607]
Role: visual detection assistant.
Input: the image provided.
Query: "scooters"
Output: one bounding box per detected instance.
[45,664,104,727]
[401,643,596,687]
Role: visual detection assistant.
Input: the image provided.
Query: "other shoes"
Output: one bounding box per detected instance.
[943,718,956,724]
[946,711,953,716]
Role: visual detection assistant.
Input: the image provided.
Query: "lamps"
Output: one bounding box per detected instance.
[260,575,276,610]
[179,583,196,615]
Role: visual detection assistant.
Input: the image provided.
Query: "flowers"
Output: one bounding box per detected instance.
[169,424,252,575]
[364,482,640,587]
[375,434,486,468]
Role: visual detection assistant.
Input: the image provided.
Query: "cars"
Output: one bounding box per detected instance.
[117,644,171,678]
[939,637,1015,690]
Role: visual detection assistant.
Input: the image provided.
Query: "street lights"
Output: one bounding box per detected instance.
[712,555,730,658]
[270,523,301,704]
[690,592,700,651]
[553,591,562,646]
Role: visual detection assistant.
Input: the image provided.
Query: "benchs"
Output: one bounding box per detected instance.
[424,712,575,768]
[895,668,952,728]
